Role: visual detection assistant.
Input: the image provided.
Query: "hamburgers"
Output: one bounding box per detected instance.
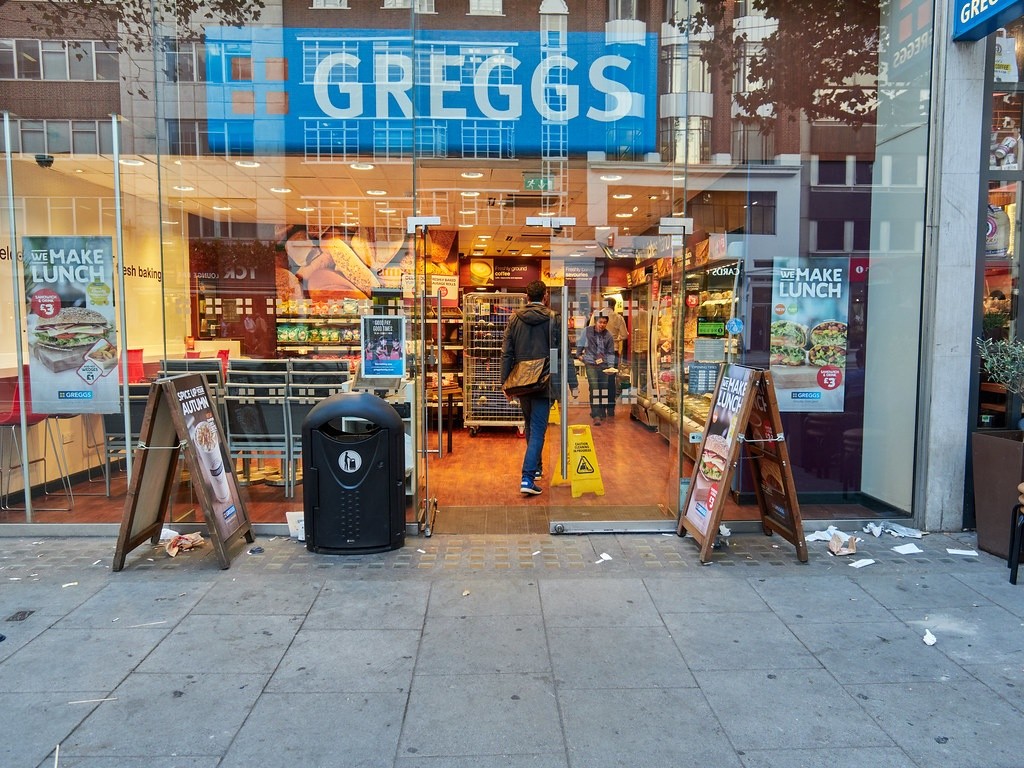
[34,308,108,348]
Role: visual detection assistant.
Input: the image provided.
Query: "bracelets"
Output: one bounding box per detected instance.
[571,387,578,390]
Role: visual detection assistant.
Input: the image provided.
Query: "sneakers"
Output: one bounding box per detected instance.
[534,469,544,480]
[519,480,543,494]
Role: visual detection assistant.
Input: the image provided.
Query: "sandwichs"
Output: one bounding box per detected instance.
[770,321,807,366]
[809,321,848,368]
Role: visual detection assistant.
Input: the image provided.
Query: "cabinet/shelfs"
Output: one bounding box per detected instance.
[276,318,465,424]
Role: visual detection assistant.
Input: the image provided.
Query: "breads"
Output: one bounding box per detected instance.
[273,223,459,314]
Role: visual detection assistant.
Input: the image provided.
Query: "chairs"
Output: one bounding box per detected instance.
[0,345,230,511]
[100,358,351,499]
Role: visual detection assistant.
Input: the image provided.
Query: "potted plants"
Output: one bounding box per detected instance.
[972,334,1024,564]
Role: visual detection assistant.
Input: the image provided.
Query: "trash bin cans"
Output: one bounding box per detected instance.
[301,392,406,555]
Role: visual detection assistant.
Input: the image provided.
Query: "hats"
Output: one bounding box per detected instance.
[594,308,608,316]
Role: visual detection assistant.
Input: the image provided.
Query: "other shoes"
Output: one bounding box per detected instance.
[607,410,615,416]
[594,417,601,425]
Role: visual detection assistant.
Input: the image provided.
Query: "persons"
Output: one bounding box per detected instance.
[751,318,763,348]
[365,335,402,361]
[576,297,629,425]
[499,279,580,495]
[856,340,865,370]
[220,310,273,355]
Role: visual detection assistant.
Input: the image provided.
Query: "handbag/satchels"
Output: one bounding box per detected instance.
[501,357,550,396]
